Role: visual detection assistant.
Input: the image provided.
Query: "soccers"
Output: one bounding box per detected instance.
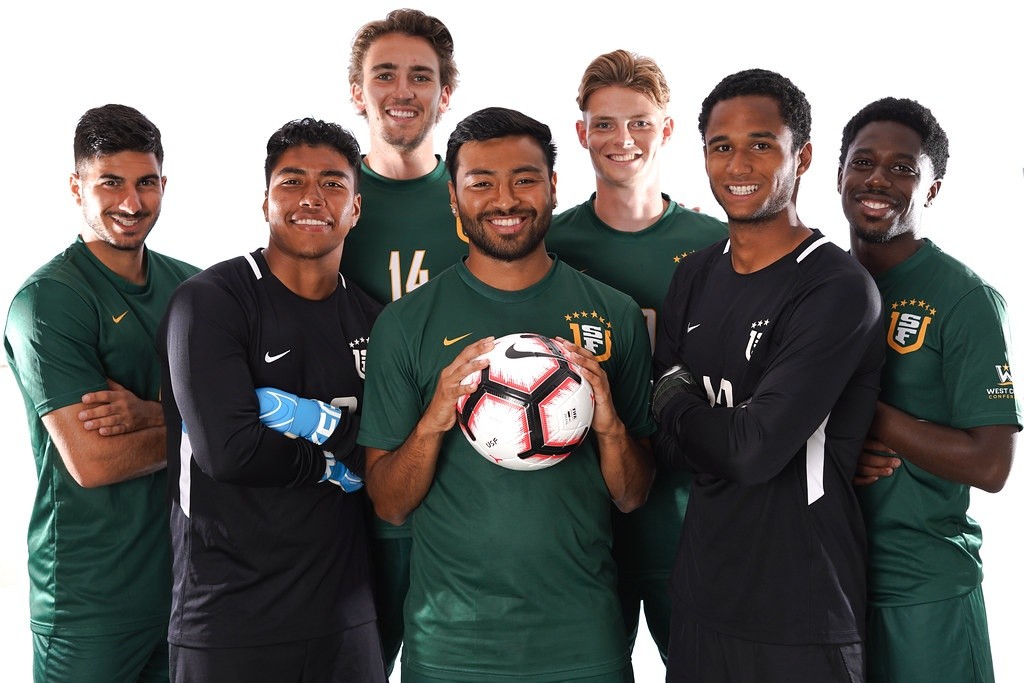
[455,333,596,472]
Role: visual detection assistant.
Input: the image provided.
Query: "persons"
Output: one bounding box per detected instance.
[834,94,1024,681]
[163,118,394,683]
[545,51,730,681]
[648,69,888,683]
[339,10,475,305]
[355,106,655,682]
[0,103,207,681]
